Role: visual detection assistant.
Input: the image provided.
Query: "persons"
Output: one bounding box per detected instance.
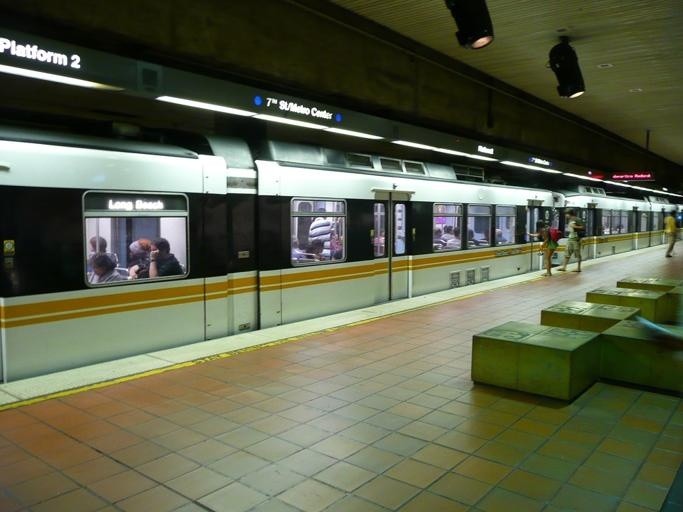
[557,209,584,272]
[604,225,624,235]
[290,209,343,261]
[88,236,184,284]
[433,226,507,249]
[529,219,561,276]
[664,208,678,258]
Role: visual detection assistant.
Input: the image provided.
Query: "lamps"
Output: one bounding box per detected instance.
[444,0,494,50]
[547,36,587,101]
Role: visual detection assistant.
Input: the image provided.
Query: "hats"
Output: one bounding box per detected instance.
[129,239,151,255]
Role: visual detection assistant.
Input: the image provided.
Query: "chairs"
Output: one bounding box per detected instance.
[88,251,186,284]
[433,239,508,252]
[295,252,317,261]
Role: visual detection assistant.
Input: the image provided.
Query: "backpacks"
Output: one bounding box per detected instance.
[548,226,562,243]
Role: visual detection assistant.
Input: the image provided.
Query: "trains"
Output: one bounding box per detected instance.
[0,105,682,383]
